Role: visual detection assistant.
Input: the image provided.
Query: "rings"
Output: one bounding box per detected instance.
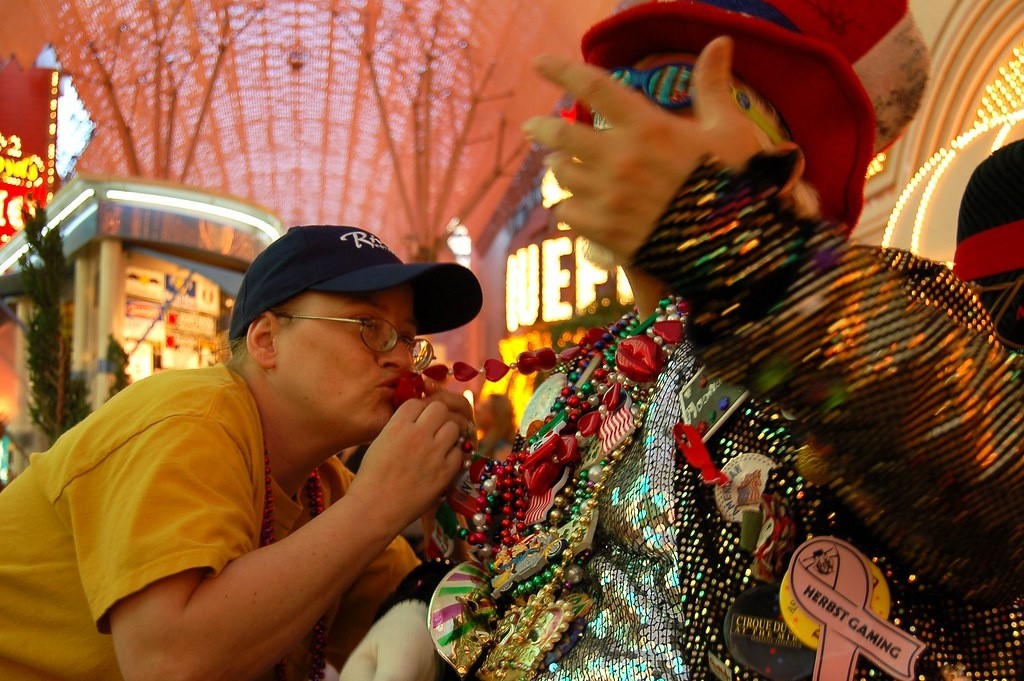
[468,423,475,436]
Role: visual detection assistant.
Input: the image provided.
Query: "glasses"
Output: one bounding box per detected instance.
[268,305,438,371]
[592,61,783,146]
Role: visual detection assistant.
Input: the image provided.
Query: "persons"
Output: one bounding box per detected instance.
[0,224,485,681]
[475,394,518,461]
[403,0,1024,681]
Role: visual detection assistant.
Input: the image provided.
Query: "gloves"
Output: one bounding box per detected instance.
[338,599,441,681]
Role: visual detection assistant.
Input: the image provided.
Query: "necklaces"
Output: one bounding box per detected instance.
[264,437,324,681]
[395,298,682,681]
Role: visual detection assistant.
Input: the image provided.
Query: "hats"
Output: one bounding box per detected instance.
[227,225,483,341]
[953,138,1024,282]
[582,0,933,240]
